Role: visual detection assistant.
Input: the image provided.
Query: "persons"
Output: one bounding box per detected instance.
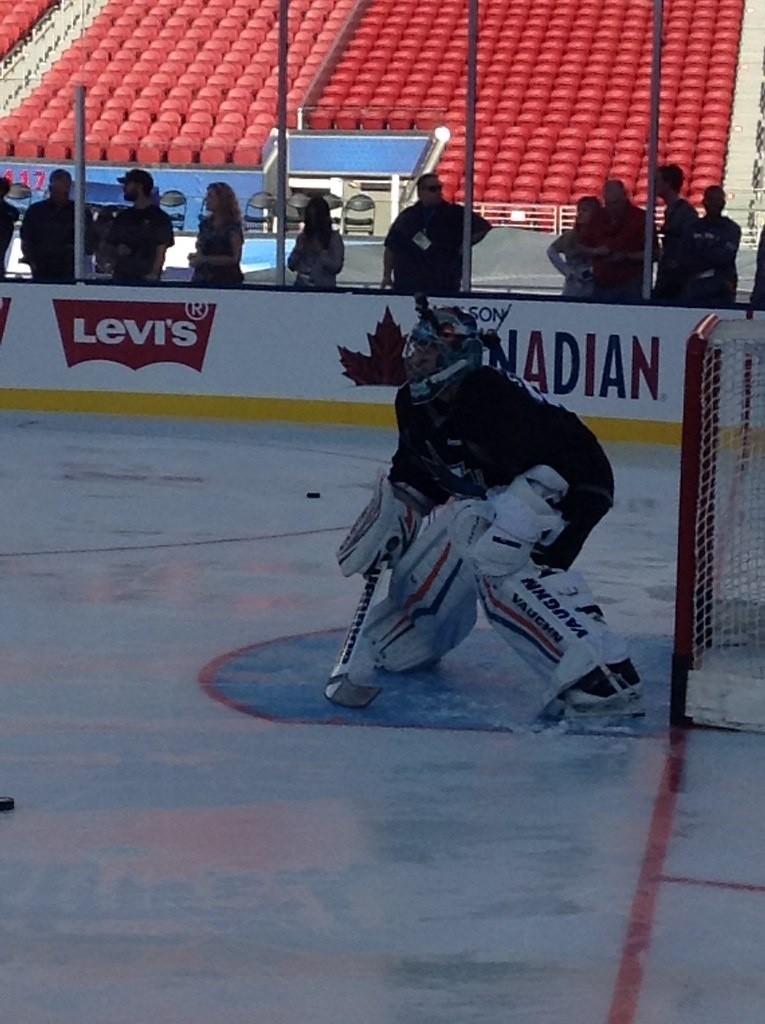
[380,174,491,292]
[19,169,96,281]
[288,196,344,289]
[337,307,648,719]
[187,182,245,282]
[96,169,175,281]
[0,178,19,277]
[547,164,742,309]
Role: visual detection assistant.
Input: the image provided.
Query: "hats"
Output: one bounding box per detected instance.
[116,169,153,189]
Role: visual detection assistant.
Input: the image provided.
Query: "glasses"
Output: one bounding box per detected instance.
[209,182,227,188]
[421,184,442,193]
[50,187,69,197]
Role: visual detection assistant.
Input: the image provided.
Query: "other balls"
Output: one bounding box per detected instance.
[306,492,321,498]
[0,796,15,811]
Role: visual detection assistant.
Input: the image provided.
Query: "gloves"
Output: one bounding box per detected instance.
[472,484,569,578]
[334,466,424,581]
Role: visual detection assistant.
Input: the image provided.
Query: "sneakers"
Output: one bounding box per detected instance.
[563,658,645,703]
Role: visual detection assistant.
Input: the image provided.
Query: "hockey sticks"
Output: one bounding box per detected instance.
[322,566,384,711]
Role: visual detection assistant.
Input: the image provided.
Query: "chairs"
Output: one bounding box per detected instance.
[0,0,745,239]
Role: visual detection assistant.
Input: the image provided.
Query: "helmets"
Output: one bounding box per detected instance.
[400,306,485,406]
